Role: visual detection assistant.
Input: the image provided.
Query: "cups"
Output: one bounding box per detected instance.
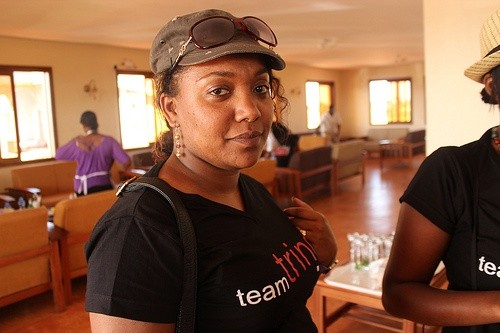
[346,230,396,272]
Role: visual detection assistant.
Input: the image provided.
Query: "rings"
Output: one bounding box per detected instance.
[300,230,306,235]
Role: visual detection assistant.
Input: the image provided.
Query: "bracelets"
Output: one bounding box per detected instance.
[321,259,338,276]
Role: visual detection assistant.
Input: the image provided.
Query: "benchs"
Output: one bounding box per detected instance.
[238,139,367,206]
[0,188,122,311]
[376,129,426,170]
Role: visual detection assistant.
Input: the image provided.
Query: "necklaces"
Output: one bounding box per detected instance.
[491,127,500,147]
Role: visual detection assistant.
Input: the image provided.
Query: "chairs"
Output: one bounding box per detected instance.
[132,152,156,172]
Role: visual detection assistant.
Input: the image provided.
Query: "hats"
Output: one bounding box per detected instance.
[150,9,286,74]
[464,10,500,83]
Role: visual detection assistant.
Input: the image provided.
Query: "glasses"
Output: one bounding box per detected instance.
[165,16,277,73]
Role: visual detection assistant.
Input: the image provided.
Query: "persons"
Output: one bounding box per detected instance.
[264,105,342,168]
[84,10,338,333]
[381,11,500,333]
[55,110,132,197]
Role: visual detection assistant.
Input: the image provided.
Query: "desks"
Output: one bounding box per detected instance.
[315,253,447,333]
